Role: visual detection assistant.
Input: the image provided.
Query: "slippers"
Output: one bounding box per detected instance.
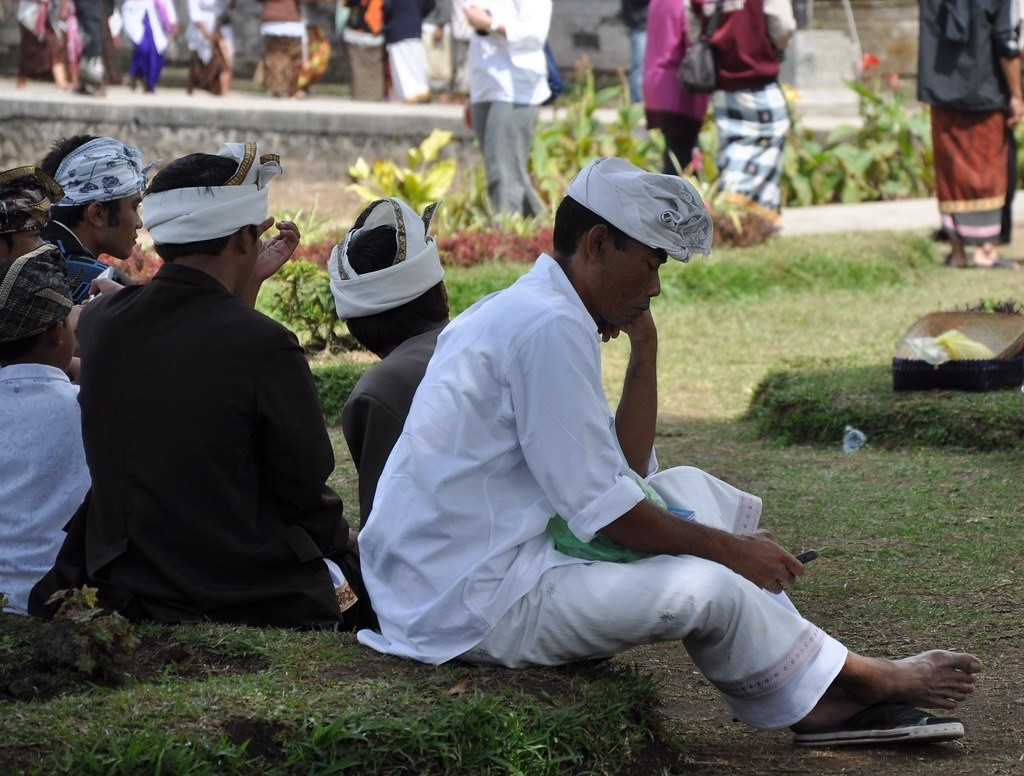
[793,703,965,746]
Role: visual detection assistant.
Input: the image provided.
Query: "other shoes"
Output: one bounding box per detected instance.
[946,253,967,267]
[973,255,1021,269]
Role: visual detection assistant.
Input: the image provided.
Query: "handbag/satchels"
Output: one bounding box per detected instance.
[543,44,563,104]
[677,41,716,94]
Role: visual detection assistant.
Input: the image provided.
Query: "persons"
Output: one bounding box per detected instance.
[0,136,163,613]
[342,1,443,99]
[358,158,982,744]
[15,0,117,96]
[619,0,797,215]
[108,0,179,92]
[29,142,359,626]
[431,0,554,228]
[328,199,450,627]
[917,0,1024,269]
[256,1,314,98]
[184,0,236,95]
